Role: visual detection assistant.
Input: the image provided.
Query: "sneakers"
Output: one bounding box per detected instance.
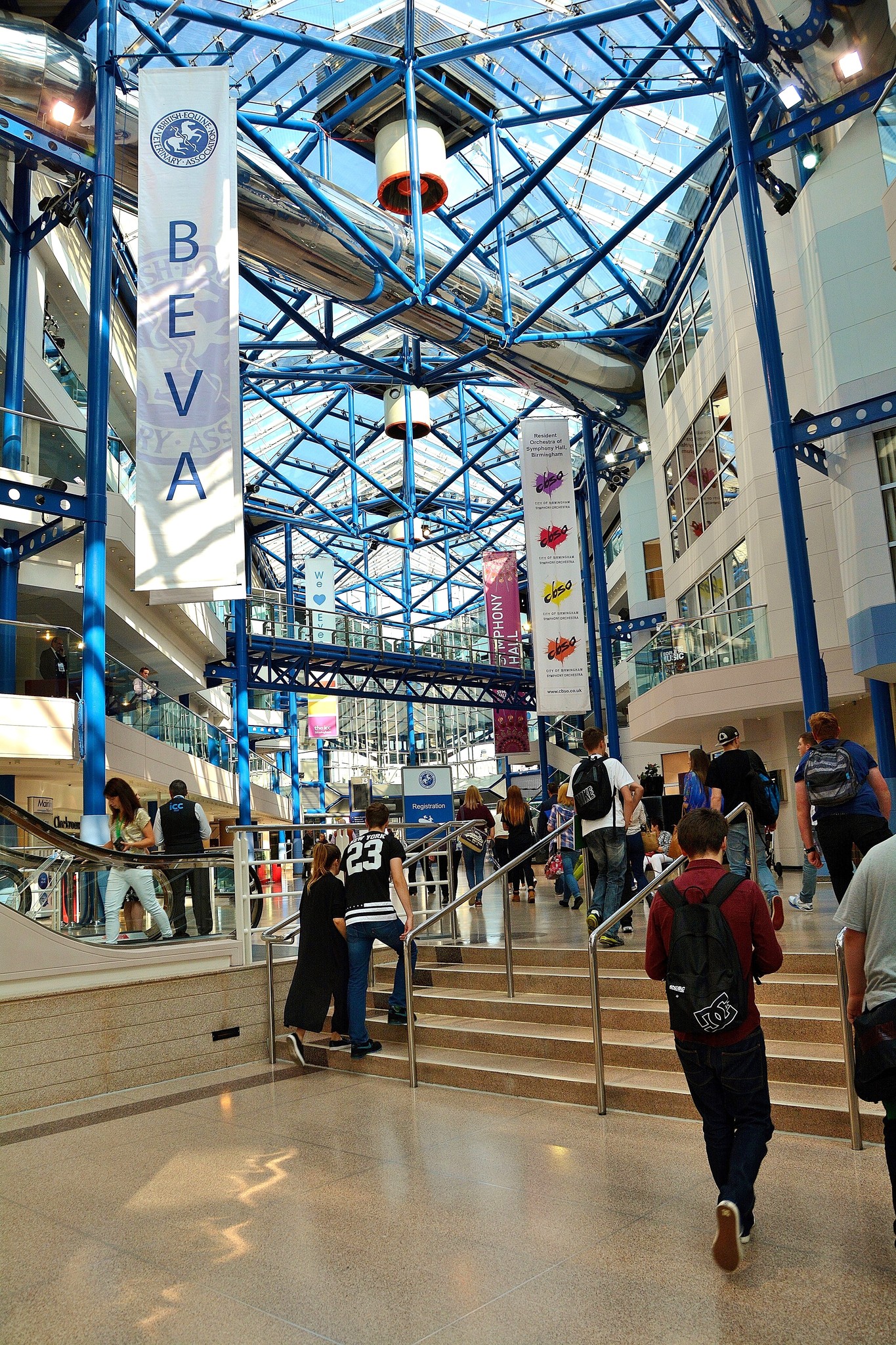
[509,887,513,894]
[475,900,482,907]
[533,878,537,889]
[329,1036,351,1051]
[559,900,569,907]
[788,894,813,912]
[351,1038,383,1059]
[740,1210,755,1243]
[621,923,634,933]
[586,913,602,936]
[468,893,476,906]
[711,1199,742,1274]
[770,895,784,931]
[441,897,449,906]
[519,886,526,890]
[286,1033,306,1067]
[599,933,625,946]
[571,896,583,910]
[388,1006,417,1025]
[555,893,563,897]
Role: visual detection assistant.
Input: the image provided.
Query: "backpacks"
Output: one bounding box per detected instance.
[803,739,868,808]
[571,757,618,821]
[657,872,751,1036]
[745,749,781,826]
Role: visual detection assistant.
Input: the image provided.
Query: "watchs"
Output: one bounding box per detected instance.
[804,845,816,856]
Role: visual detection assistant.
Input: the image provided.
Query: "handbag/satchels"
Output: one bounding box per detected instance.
[460,805,490,852]
[303,848,313,858]
[640,824,659,853]
[544,854,564,880]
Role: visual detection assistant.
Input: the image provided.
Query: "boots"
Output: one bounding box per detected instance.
[512,891,520,902]
[527,889,535,903]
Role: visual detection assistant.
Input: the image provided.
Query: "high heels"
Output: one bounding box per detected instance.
[409,892,417,896]
[428,887,436,895]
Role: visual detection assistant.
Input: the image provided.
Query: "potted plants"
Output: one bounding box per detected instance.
[637,762,664,797]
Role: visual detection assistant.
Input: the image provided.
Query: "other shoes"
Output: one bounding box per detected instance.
[302,876,308,878]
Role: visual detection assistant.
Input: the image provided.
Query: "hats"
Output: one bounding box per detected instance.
[715,726,739,747]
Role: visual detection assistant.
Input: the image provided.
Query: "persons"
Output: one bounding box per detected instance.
[634,818,676,889]
[76,777,174,945]
[152,779,214,939]
[299,818,462,906]
[832,833,896,1251]
[281,842,352,1067]
[456,785,494,907]
[133,667,157,733]
[794,712,893,910]
[39,636,69,679]
[683,748,725,817]
[339,803,418,1060]
[566,727,636,946]
[788,732,857,913]
[488,784,654,932]
[708,725,786,931]
[644,808,785,1276]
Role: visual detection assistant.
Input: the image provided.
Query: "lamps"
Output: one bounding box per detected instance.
[638,441,649,453]
[777,84,805,111]
[802,143,824,169]
[612,473,621,483]
[51,93,83,126]
[604,452,618,463]
[608,483,617,493]
[838,50,863,78]
[616,466,630,474]
